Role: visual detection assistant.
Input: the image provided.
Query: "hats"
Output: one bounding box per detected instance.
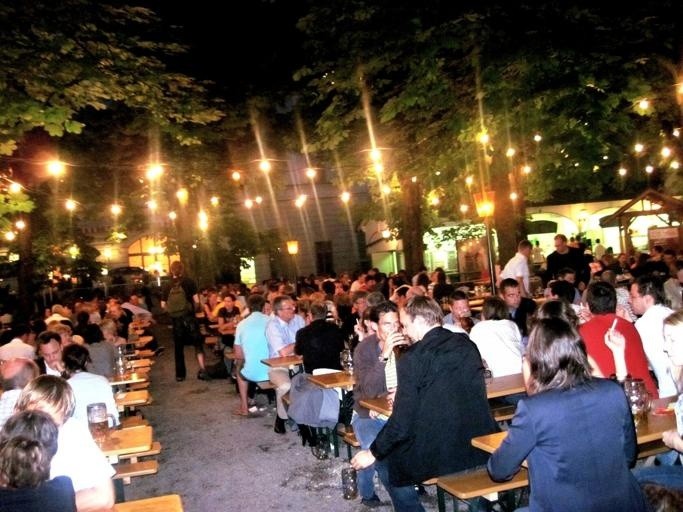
[44,313,72,326]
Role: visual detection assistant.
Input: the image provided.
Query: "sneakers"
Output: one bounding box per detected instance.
[198,369,211,381]
[247,394,260,406]
[361,492,381,506]
[155,346,166,360]
[176,377,184,382]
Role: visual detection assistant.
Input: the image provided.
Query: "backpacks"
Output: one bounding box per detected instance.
[165,278,191,318]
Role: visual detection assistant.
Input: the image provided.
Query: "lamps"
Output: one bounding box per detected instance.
[286,240,299,255]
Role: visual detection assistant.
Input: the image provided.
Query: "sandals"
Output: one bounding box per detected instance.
[231,409,249,416]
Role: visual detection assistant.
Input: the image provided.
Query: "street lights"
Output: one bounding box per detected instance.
[284,235,300,281]
[474,190,499,296]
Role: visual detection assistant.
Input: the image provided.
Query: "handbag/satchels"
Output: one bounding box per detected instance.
[337,390,360,427]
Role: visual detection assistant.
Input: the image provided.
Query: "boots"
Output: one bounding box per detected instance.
[274,413,288,435]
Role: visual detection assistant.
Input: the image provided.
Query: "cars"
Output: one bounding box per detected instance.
[0,260,18,293]
[111,266,150,285]
[72,258,108,276]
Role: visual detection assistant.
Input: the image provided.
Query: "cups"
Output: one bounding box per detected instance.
[338,350,354,374]
[233,313,243,330]
[109,342,129,376]
[84,402,117,445]
[623,378,656,422]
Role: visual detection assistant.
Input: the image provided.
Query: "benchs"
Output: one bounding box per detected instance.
[72,317,184,511]
[195,271,682,509]
